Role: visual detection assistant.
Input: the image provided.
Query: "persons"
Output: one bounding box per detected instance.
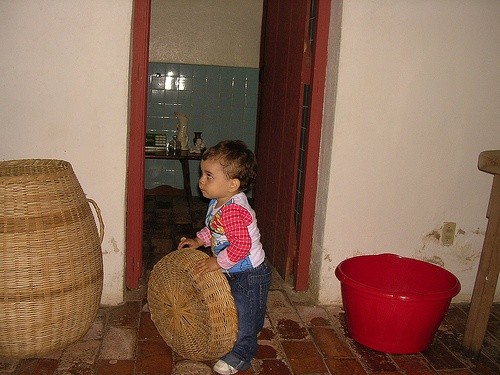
[177,140,272,375]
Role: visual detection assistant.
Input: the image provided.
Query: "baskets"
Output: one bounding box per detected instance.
[0,158,104,361]
[147,248,238,361]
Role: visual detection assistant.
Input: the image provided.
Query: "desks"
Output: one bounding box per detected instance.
[146,150,204,229]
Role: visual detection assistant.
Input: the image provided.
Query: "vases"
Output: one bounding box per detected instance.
[193,131,202,147]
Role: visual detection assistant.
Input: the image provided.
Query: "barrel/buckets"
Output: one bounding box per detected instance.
[334,253,461,354]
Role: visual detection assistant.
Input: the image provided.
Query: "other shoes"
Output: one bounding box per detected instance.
[211,359,238,375]
